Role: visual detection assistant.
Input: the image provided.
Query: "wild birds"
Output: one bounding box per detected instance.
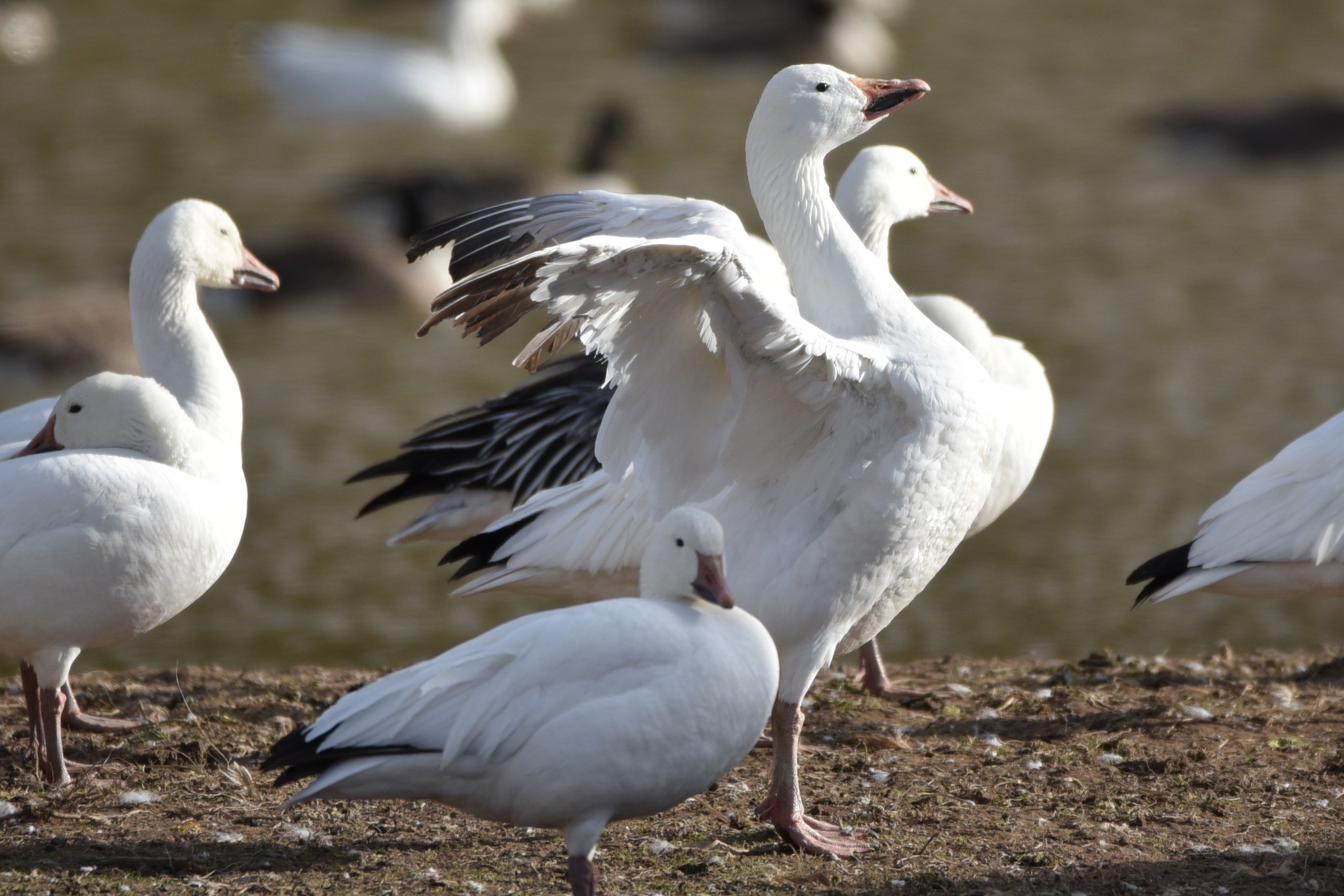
[2,197,281,792]
[257,61,1054,896]
[1127,408,1343,607]
